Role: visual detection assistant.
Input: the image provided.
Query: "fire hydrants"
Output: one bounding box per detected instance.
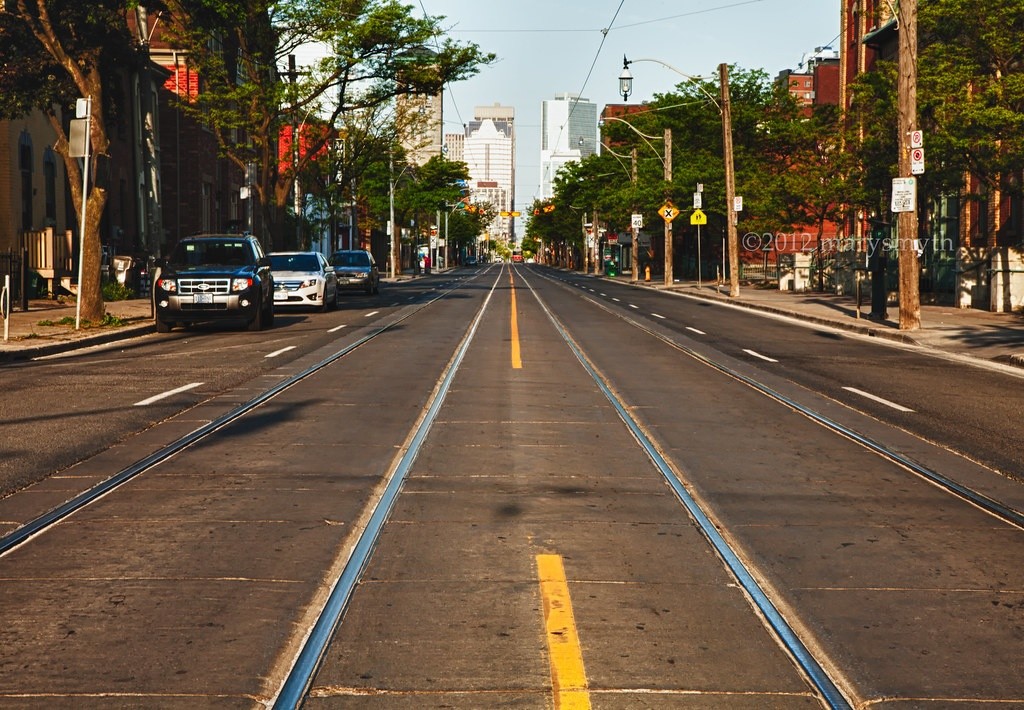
[644,265,652,282]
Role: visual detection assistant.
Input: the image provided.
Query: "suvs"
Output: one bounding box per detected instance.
[153,231,276,332]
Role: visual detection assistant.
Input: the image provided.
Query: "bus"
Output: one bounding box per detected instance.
[512,249,524,263]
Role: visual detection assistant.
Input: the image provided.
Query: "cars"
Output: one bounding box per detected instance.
[265,252,340,312]
[327,249,381,295]
[465,256,478,266]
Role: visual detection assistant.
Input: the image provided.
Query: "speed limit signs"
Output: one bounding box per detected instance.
[631,214,644,229]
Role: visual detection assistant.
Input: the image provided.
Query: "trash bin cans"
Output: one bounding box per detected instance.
[109,255,145,297]
[606,261,617,277]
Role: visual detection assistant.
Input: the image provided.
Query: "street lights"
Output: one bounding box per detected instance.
[598,117,673,287]
[385,142,446,277]
[293,97,354,252]
[578,136,639,284]
[619,54,742,298]
